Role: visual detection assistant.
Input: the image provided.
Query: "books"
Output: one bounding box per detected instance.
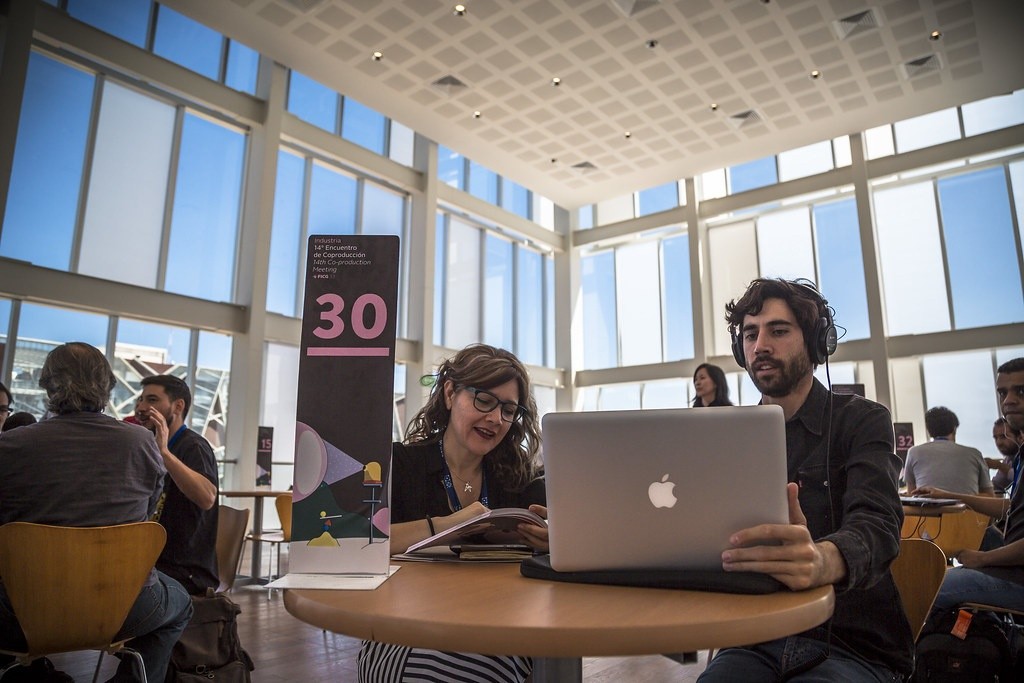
[403,507,548,553]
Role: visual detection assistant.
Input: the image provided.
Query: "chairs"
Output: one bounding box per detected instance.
[900,503,992,554]
[890,539,947,642]
[216,504,250,594]
[0,522,168,683]
[238,493,292,601]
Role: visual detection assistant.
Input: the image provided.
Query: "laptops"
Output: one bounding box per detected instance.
[899,496,958,507]
[543,403,790,573]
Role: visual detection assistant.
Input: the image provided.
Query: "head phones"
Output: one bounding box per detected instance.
[730,281,837,371]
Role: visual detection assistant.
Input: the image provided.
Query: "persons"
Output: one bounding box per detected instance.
[694,363,733,406]
[693,278,915,683]
[390,346,549,554]
[902,407,995,501]
[0,381,12,431]
[2,412,37,432]
[911,359,1024,611]
[123,395,141,425]
[137,375,220,596]
[0,340,192,683]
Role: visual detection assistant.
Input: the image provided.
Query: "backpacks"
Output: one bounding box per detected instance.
[911,606,1024,683]
[164,585,255,683]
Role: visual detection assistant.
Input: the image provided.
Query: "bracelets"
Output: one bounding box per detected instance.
[426,515,436,536]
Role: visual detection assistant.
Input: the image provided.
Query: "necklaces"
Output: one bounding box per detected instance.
[450,468,481,492]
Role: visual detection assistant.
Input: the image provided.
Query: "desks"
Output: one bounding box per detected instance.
[283,560,836,683]
[219,490,291,592]
[900,494,968,518]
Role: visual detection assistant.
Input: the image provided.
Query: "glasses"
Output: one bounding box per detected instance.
[0,406,14,417]
[453,383,527,423]
